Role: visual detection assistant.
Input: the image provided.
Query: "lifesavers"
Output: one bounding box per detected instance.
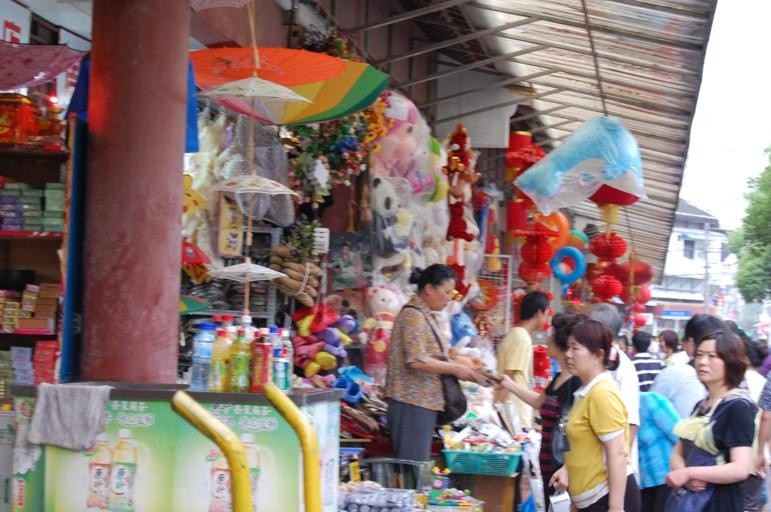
[533,210,570,250]
[548,246,585,284]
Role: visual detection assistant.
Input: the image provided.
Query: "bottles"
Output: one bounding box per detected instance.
[222,313,236,341]
[249,331,261,379]
[190,323,212,393]
[212,315,222,329]
[229,329,250,393]
[210,324,217,339]
[277,329,290,390]
[240,316,252,338]
[251,327,273,393]
[283,330,293,386]
[269,325,283,390]
[209,330,229,392]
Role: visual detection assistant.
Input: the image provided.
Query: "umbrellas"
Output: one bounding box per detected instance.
[192,71,314,165]
[188,47,392,136]
[206,259,292,315]
[210,169,302,247]
[1,39,90,92]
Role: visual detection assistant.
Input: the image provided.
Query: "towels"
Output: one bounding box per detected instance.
[27,382,114,452]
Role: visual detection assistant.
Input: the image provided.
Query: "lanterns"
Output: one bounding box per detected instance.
[587,184,641,239]
[621,253,654,285]
[507,188,535,237]
[626,303,648,314]
[516,263,553,288]
[630,328,642,338]
[519,224,553,265]
[527,344,550,382]
[591,274,624,304]
[588,230,628,263]
[501,132,549,184]
[622,313,647,328]
[621,285,651,304]
[587,261,628,285]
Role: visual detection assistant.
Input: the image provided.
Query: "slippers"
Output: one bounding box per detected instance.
[290,301,375,403]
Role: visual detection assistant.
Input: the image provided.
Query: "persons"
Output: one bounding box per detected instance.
[754,372,771,510]
[754,339,770,375]
[663,329,759,512]
[493,291,551,433]
[659,331,693,366]
[490,313,583,511]
[548,302,642,485]
[565,319,643,512]
[639,392,684,511]
[382,264,457,460]
[629,332,665,391]
[646,314,731,418]
[615,336,628,357]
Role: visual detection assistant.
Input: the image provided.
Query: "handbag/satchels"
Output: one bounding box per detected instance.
[548,487,575,512]
[404,303,467,425]
[663,397,753,512]
[551,379,573,467]
